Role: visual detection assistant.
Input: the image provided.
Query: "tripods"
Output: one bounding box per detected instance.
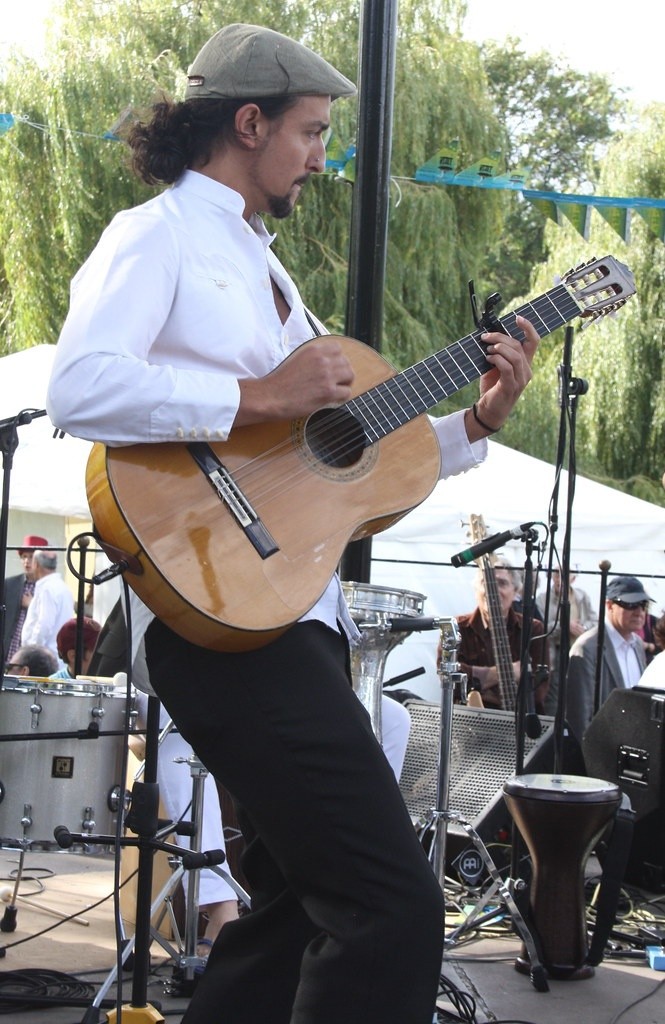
[380,615,549,995]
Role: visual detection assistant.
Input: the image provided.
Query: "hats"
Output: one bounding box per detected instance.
[606,576,657,604]
[56,616,102,657]
[18,535,48,555]
[183,23,357,100]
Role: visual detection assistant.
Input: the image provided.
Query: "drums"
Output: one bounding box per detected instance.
[340,581,428,749]
[503,773,622,982]
[0,673,136,861]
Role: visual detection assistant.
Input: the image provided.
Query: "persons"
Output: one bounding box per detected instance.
[437,554,548,716]
[536,568,599,717]
[6,646,58,677]
[22,550,75,669]
[638,615,665,691]
[566,577,658,746]
[47,617,102,678]
[118,575,412,959]
[48,25,540,1024]
[2,535,50,675]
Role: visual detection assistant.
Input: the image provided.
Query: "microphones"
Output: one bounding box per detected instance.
[523,656,541,739]
[451,522,534,568]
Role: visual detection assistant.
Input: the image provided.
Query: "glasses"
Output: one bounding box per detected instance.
[613,600,648,609]
[4,662,24,675]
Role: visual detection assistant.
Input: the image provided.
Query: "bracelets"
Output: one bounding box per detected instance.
[473,404,502,433]
[581,625,587,630]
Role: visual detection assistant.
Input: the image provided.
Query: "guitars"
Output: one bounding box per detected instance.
[84,251,639,652]
[459,509,522,712]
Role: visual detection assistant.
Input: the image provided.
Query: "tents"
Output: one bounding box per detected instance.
[0,340,665,706]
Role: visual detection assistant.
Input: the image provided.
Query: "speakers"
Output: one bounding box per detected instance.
[395,698,584,889]
[580,685,665,888]
